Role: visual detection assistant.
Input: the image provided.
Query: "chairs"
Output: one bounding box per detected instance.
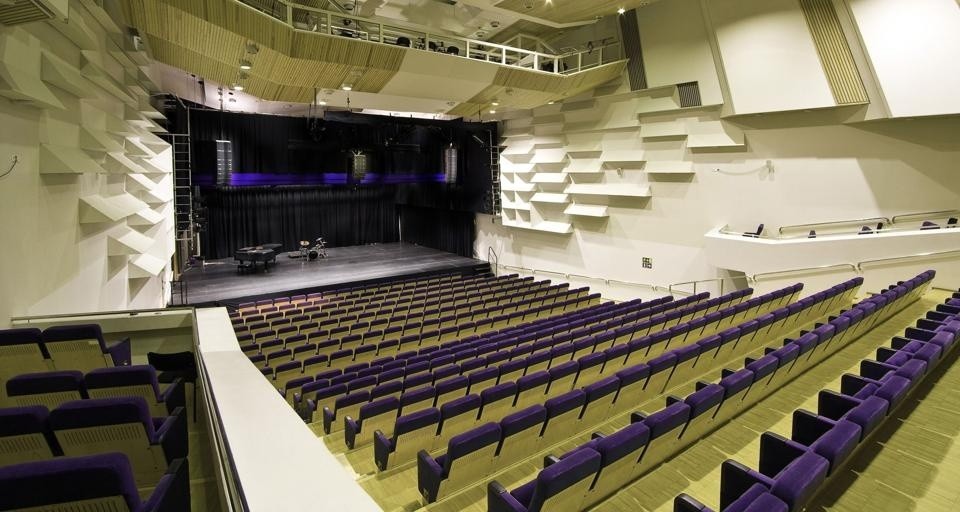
[232,269,960,511]
[5,369,85,409]
[39,324,131,372]
[0,450,187,512]
[745,224,763,238]
[81,366,186,417]
[46,398,189,504]
[0,328,49,407]
[0,406,64,467]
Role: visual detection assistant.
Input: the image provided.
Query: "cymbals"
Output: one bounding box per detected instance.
[300,241,309,245]
[316,238,322,242]
[321,242,327,243]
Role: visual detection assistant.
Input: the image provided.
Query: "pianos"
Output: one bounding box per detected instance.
[234,244,283,269]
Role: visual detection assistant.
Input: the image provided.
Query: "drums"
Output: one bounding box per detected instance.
[309,251,318,259]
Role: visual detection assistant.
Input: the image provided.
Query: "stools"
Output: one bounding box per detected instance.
[237,264,251,273]
